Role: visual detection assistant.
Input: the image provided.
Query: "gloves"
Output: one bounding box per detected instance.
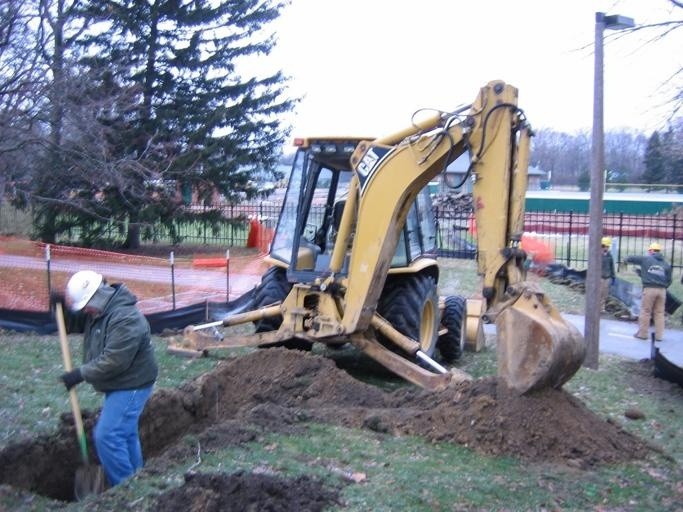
[59,367,84,392]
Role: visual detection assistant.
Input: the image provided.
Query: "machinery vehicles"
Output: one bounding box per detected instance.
[166,78,586,396]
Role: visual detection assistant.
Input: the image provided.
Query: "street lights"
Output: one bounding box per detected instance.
[581,10,636,371]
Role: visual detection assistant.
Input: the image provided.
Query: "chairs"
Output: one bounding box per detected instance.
[328,201,354,249]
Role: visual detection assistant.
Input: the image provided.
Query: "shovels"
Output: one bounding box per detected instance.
[54,302,105,503]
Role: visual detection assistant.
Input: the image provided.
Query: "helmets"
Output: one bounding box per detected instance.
[600,237,613,248]
[63,270,103,313]
[647,242,662,251]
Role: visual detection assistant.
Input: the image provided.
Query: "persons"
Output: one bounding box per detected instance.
[601,236,673,343]
[48,269,158,487]
